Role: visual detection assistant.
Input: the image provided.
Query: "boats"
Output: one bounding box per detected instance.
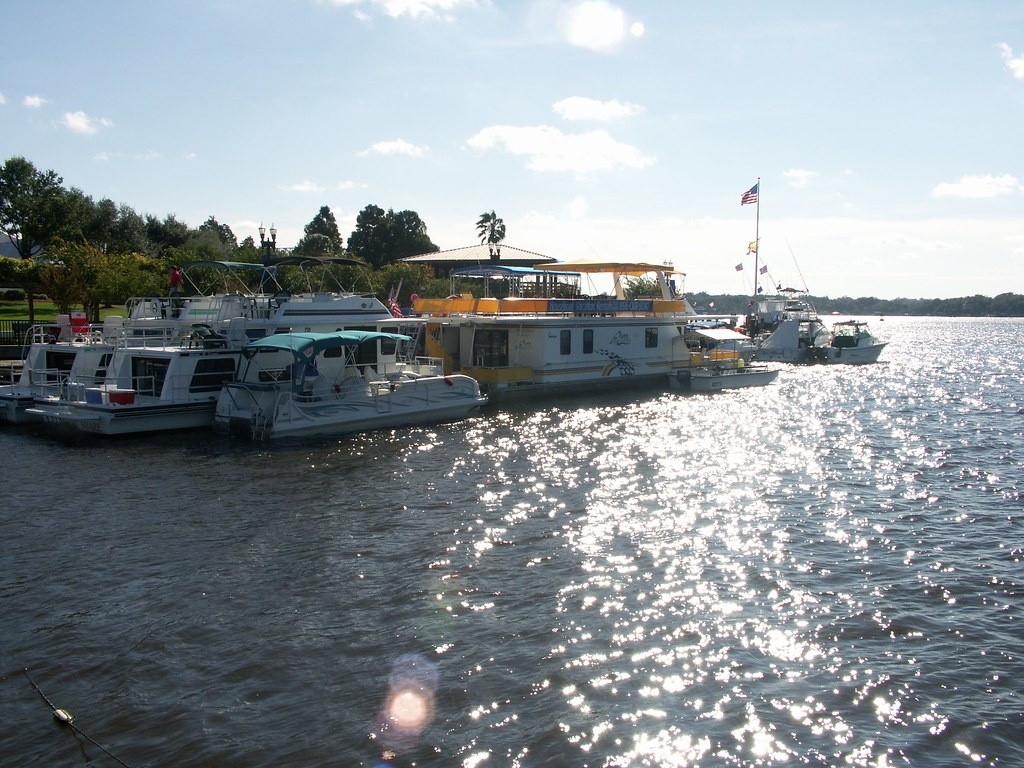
[0,176,890,444]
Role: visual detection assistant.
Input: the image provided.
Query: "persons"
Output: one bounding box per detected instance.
[168,265,182,318]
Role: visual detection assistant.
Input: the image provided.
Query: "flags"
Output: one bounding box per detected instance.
[741,184,757,206]
[735,263,743,271]
[759,265,768,274]
[758,287,763,293]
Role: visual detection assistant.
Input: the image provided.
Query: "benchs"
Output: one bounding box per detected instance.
[188,323,228,350]
[217,316,250,348]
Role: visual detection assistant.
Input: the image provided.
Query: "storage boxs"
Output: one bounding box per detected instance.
[110,387,137,405]
[85,387,102,403]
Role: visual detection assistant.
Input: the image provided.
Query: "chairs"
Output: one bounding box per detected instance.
[313,366,412,402]
[94,315,124,345]
[69,310,93,345]
